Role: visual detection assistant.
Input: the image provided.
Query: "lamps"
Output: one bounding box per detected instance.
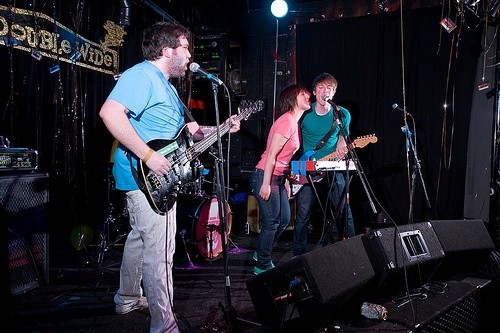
[439,0,458,33]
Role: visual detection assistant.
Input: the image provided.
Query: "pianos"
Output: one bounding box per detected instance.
[292,157,358,247]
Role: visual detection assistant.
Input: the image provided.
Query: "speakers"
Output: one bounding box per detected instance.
[0,173,50,301]
[246,235,375,332]
[362,220,444,300]
[429,217,495,277]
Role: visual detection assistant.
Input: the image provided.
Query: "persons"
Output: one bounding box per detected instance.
[288,73,356,275]
[252,83,311,275]
[98,21,241,333]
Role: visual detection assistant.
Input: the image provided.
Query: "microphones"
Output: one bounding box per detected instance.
[391,103,411,117]
[189,62,223,85]
[322,93,340,111]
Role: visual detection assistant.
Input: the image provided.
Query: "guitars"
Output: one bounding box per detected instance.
[137,99,265,216]
[291,133,378,196]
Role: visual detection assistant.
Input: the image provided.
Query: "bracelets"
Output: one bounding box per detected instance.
[142,148,154,163]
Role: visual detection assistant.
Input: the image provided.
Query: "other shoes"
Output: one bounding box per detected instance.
[253,250,276,268]
[253,266,268,275]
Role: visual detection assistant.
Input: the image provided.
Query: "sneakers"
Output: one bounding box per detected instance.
[115,295,149,314]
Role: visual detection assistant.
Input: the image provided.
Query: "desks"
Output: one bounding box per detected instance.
[246,195,297,233]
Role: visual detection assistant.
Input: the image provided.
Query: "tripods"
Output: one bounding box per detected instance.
[98,164,124,269]
[199,86,262,332]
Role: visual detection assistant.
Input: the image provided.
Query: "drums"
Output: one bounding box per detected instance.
[177,193,232,262]
